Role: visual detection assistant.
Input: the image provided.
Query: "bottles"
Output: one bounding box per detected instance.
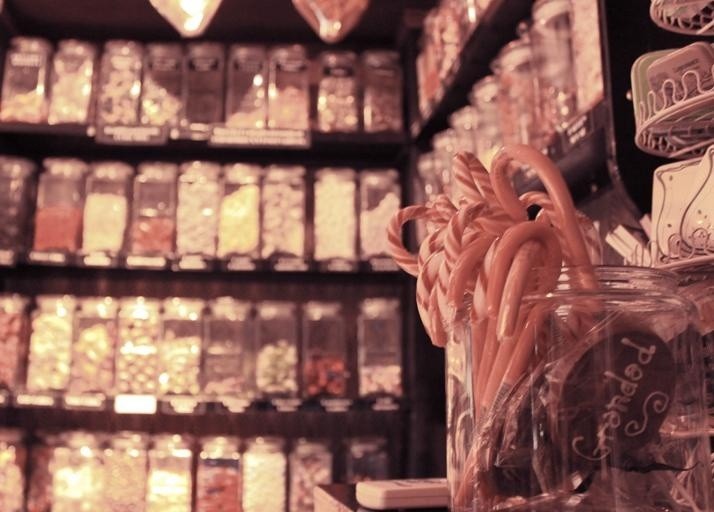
[0,281,405,409]
[3,33,405,139]
[409,1,614,253]
[0,415,391,511]
[442,263,714,512]
[2,149,404,257]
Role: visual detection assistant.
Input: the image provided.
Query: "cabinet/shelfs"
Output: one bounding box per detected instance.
[0,1,693,480]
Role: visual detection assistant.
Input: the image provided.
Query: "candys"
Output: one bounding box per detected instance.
[385,146,714,512]
